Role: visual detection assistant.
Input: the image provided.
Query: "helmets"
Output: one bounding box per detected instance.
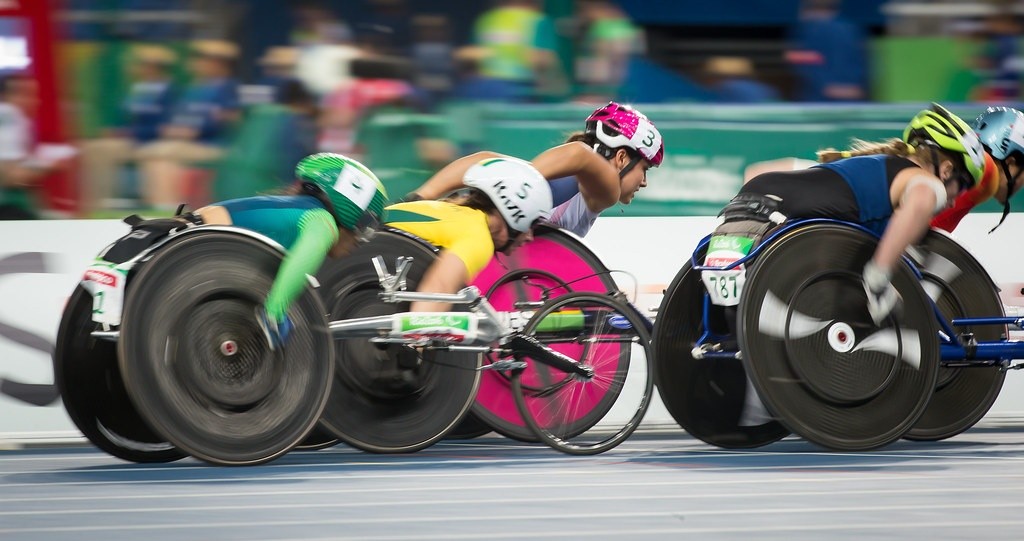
[295,153,388,231]
[902,102,986,191]
[973,107,1024,160]
[585,102,663,167]
[462,157,553,232]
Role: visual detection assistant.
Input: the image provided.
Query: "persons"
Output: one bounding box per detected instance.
[392,97,665,239]
[376,151,557,342]
[0,1,1024,218]
[708,100,1023,322]
[83,149,389,351]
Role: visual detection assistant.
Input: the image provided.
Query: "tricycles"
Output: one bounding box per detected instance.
[51,221,1024,466]
